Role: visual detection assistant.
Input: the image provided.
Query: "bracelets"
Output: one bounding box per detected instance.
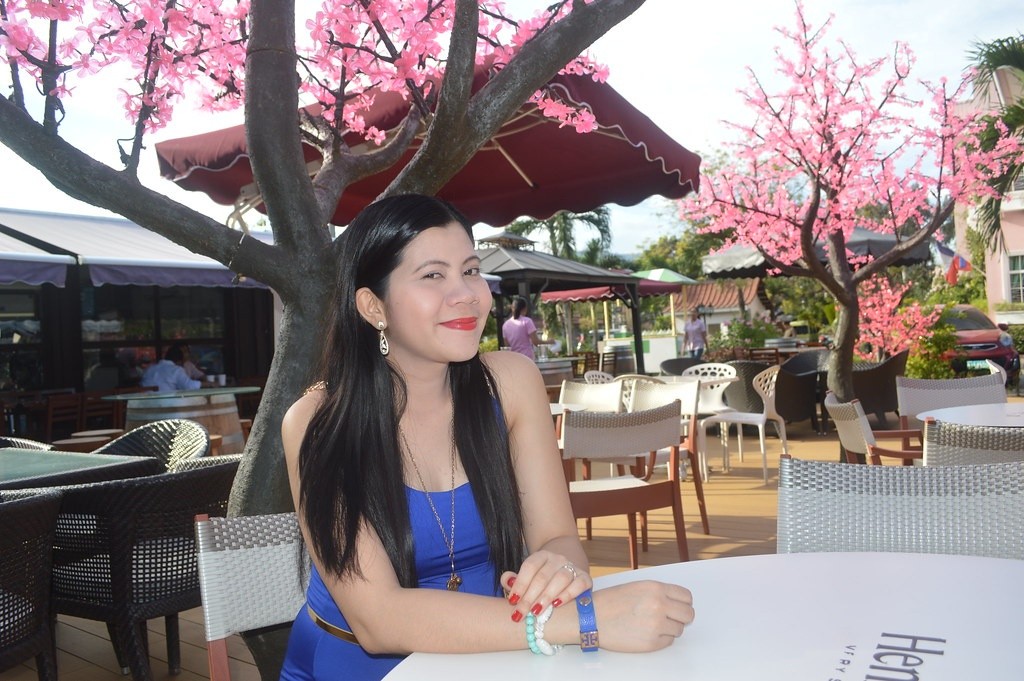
[535,603,562,656]
[575,589,599,653]
[526,611,541,655]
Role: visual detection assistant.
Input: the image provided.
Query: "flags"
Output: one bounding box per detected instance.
[932,239,972,285]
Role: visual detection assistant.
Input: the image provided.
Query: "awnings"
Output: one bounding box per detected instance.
[0,208,275,288]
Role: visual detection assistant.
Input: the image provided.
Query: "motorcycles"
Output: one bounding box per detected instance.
[819,335,834,349]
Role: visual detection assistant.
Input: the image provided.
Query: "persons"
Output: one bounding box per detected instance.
[139,348,216,393]
[84,348,152,393]
[503,296,555,361]
[782,322,795,337]
[175,343,210,380]
[681,306,709,361]
[577,333,585,352]
[278,193,694,681]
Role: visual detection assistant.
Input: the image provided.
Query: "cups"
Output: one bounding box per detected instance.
[500,347,510,351]
[537,345,549,361]
[218,374,226,386]
[207,375,214,382]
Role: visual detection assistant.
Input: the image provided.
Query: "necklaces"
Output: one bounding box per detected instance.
[395,385,463,592]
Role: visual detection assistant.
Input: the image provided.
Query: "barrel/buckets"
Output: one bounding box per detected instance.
[534,360,574,424]
[125,393,245,453]
[764,336,796,348]
[602,345,636,377]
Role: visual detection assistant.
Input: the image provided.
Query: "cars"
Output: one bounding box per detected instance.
[921,304,1021,386]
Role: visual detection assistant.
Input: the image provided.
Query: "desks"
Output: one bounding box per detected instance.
[915,403,1024,428]
[749,347,828,360]
[550,403,587,416]
[1,448,155,619]
[534,357,586,386]
[101,387,262,458]
[0,388,76,436]
[380,553,1024,681]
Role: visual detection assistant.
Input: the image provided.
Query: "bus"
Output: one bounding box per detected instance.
[789,320,825,344]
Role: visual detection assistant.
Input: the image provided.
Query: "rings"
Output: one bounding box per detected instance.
[563,565,577,580]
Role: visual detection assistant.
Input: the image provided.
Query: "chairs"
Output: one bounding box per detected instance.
[0,351,1024,681]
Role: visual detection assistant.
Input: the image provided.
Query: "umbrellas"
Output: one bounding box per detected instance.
[153,57,700,229]
[633,268,700,285]
[541,277,681,301]
[702,227,931,278]
